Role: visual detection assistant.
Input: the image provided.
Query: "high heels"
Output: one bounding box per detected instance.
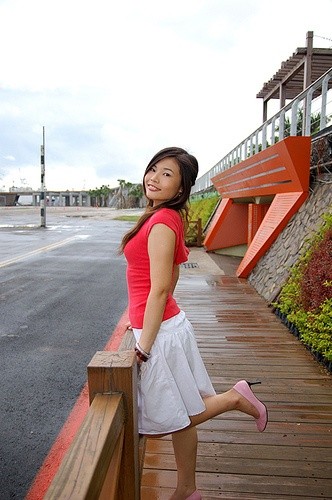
[233,378,267,432]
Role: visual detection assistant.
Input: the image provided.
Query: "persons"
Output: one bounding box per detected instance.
[115,147,268,500]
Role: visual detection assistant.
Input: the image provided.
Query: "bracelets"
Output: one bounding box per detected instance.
[135,343,150,362]
